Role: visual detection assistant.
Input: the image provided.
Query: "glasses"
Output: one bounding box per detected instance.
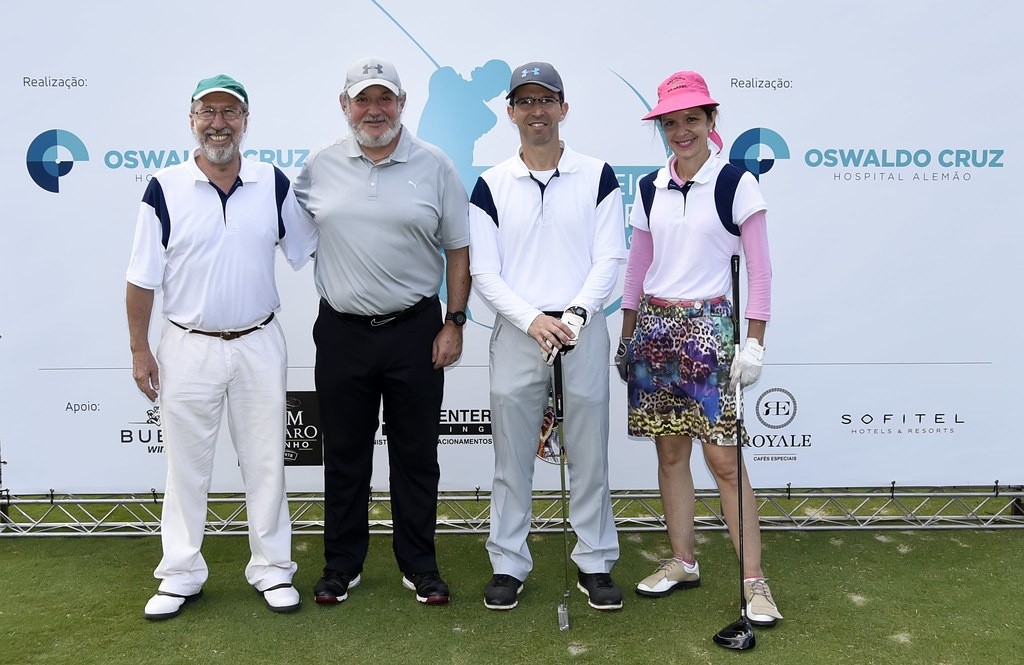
[512,98,562,107]
[192,108,246,120]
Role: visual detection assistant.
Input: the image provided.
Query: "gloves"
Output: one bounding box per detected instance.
[729,337,765,392]
[615,336,633,384]
[540,309,583,366]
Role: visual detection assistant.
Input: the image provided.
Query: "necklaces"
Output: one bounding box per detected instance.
[373,152,391,162]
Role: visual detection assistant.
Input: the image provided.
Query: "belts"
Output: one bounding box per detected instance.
[543,309,564,318]
[321,296,436,327]
[168,312,274,340]
[648,295,726,308]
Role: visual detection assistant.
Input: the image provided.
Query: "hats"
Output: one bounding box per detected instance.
[344,59,401,99]
[506,62,564,100]
[191,74,248,105]
[641,71,720,121]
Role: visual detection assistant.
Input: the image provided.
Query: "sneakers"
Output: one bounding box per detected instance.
[483,574,523,609]
[314,570,361,602]
[744,578,783,625]
[402,571,450,604]
[577,569,622,609]
[637,557,701,596]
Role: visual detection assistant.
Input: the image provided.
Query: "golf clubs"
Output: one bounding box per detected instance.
[554,353,570,632]
[712,254,756,651]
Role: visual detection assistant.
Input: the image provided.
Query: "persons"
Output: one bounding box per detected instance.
[126,75,319,622]
[614,71,785,628]
[292,58,472,604]
[468,61,627,611]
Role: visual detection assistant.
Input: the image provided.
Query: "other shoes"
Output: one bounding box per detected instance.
[144,588,203,618]
[262,583,300,611]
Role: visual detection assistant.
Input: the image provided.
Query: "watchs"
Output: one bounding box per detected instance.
[445,311,467,326]
[570,307,587,325]
